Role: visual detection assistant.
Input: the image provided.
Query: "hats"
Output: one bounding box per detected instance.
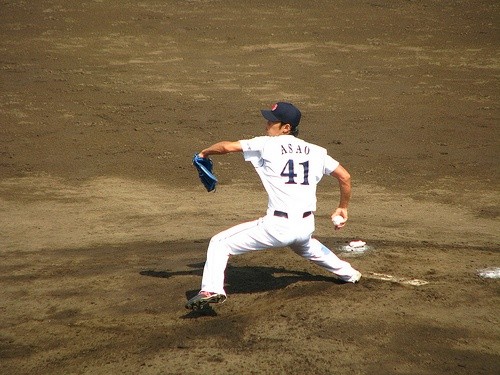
[260,102,301,127]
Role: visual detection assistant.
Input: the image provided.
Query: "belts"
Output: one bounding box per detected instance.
[274,210,312,219]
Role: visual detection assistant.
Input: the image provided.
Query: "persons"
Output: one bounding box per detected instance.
[185,101,361,311]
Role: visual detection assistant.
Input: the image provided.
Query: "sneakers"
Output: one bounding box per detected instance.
[184,291,226,311]
[333,273,362,284]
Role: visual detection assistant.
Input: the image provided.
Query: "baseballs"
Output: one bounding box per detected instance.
[332,215,344,226]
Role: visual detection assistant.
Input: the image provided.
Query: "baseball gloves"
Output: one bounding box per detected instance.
[191,153,218,192]
